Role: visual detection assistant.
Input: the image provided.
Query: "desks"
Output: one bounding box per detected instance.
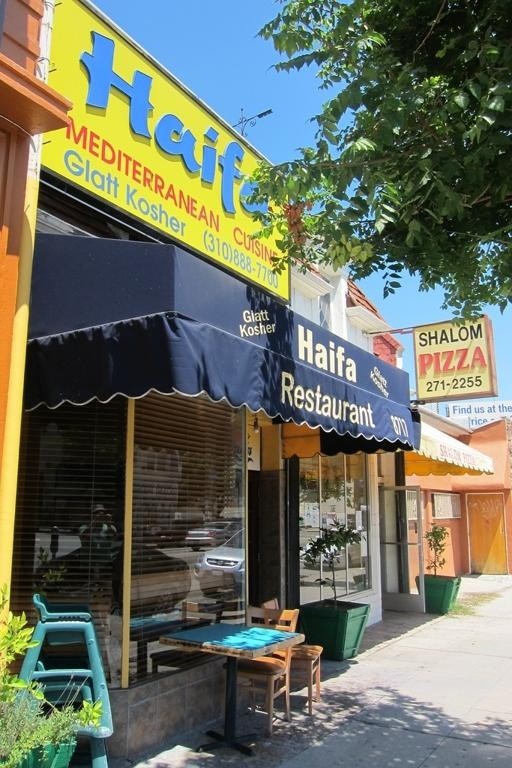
[123,618,176,685]
[159,623,307,758]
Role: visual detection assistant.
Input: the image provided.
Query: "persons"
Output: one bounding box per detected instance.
[80,505,119,635]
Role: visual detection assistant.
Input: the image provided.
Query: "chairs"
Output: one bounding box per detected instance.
[219,601,245,624]
[234,603,299,742]
[261,595,325,723]
[149,599,224,677]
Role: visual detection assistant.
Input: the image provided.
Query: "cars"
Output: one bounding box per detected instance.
[34,541,192,615]
[132,523,176,546]
[300,528,365,569]
[184,521,242,550]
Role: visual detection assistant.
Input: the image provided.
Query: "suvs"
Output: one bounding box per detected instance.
[193,529,245,594]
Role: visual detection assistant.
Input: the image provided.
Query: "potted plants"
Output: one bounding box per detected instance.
[297,521,372,663]
[415,520,462,615]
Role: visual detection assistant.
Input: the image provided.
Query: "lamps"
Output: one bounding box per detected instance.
[253,410,260,437]
